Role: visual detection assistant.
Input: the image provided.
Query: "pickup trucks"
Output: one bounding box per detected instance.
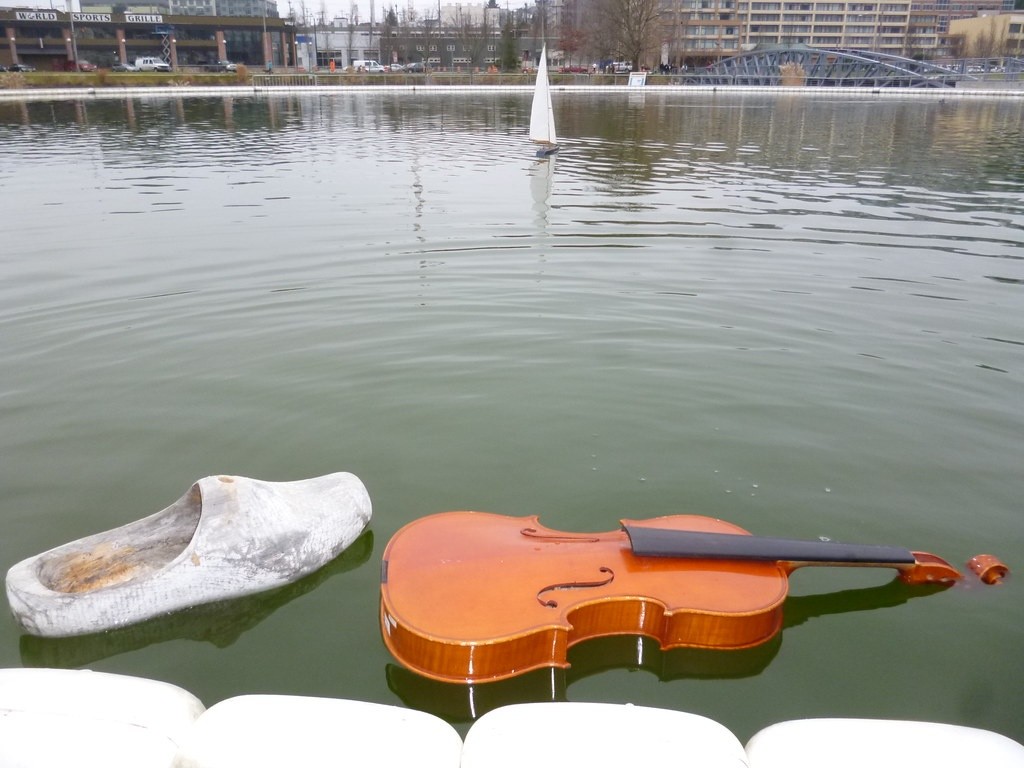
[136,57,171,73]
[203,61,237,74]
[606,62,633,73]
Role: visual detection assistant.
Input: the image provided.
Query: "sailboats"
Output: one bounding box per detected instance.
[527,42,559,159]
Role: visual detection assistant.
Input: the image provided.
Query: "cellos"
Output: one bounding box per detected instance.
[377,508,1011,684]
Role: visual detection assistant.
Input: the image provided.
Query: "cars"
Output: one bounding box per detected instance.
[112,63,141,72]
[397,62,427,74]
[385,64,402,72]
[66,59,97,73]
[8,64,37,72]
[522,67,532,74]
[561,65,593,73]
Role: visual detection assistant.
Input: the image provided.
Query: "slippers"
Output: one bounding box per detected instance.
[4,472,373,639]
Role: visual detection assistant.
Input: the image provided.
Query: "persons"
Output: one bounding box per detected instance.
[660,62,688,75]
[267,60,273,75]
[608,65,616,74]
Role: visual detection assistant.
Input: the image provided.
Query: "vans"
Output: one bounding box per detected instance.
[353,60,384,73]
